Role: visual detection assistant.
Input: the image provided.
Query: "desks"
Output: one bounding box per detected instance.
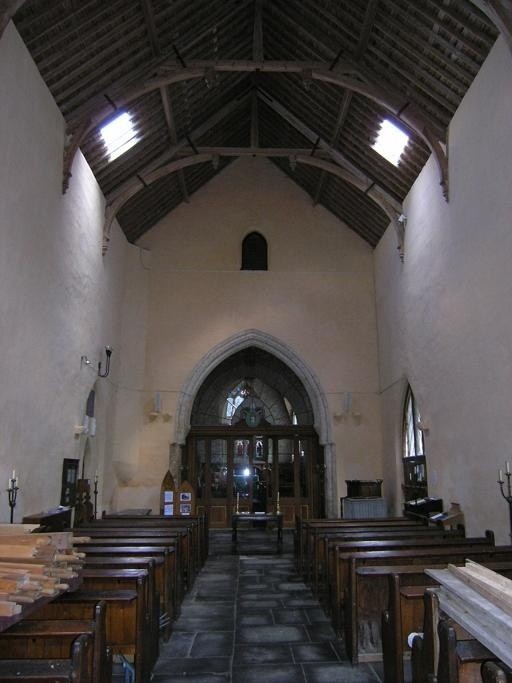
[232,514,283,554]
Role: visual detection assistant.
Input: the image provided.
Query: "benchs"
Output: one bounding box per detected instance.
[0,509,206,683]
[295,508,512,682]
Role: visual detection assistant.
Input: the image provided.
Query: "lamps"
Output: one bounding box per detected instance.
[81,345,112,377]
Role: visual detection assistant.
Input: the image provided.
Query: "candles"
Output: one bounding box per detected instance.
[95,469,97,482]
[278,491,280,501]
[8,479,11,489]
[499,470,502,481]
[13,467,15,481]
[506,461,510,472]
[237,493,239,503]
[14,477,18,487]
